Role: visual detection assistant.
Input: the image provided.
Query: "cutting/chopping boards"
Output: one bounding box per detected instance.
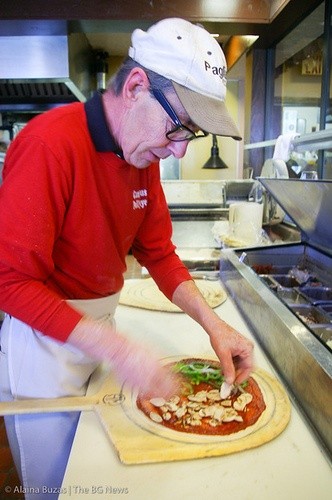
[120,276,226,312]
[0,352,292,466]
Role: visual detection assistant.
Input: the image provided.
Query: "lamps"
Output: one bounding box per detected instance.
[202,132,230,169]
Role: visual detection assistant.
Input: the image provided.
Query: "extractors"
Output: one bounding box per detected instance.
[0,3,106,114]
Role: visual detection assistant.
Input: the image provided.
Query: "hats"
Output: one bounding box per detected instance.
[129,18,242,141]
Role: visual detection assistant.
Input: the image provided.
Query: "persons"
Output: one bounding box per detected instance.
[0,15,257,500]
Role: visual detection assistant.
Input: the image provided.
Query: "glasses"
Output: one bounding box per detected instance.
[152,90,207,141]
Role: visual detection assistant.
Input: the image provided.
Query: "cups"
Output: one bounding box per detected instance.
[299,170,319,181]
[296,113,307,135]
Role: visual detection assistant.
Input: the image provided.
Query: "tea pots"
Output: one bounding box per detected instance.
[229,200,265,243]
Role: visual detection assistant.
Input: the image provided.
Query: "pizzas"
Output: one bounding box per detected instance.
[120,354,275,442]
